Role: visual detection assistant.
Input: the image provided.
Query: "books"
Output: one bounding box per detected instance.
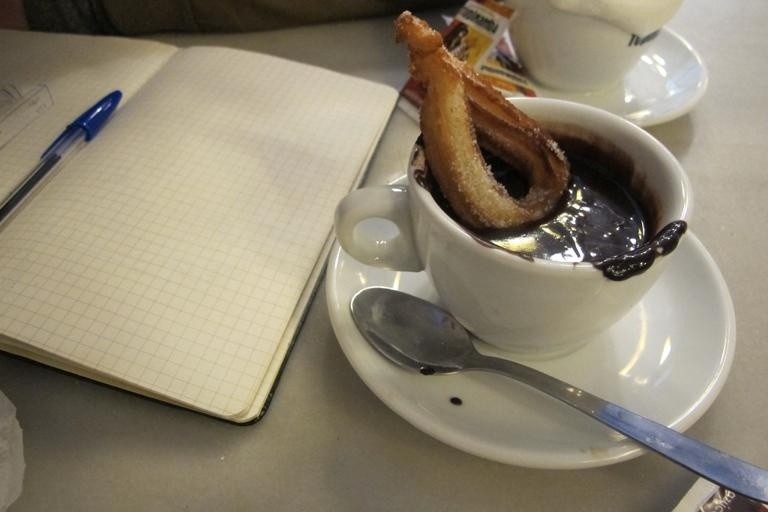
[0,25,399,428]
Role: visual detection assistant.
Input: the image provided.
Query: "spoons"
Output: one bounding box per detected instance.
[350,288,768,506]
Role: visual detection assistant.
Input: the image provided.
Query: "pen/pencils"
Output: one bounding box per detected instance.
[0,90,123,226]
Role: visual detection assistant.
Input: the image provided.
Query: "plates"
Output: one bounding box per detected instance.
[381,28,711,131]
[324,173,740,471]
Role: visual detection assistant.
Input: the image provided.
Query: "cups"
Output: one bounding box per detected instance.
[333,97,691,360]
[508,1,682,91]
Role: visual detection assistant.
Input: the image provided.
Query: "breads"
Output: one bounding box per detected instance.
[392,8,570,232]
